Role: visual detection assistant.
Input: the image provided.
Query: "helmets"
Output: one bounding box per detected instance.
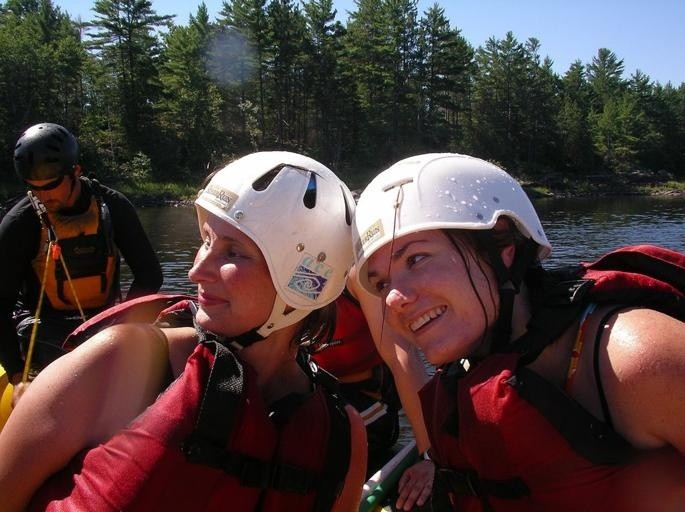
[14,123,78,180]
[351,152,553,299]
[194,152,356,310]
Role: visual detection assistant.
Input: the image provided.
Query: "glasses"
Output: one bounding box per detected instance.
[24,177,65,192]
